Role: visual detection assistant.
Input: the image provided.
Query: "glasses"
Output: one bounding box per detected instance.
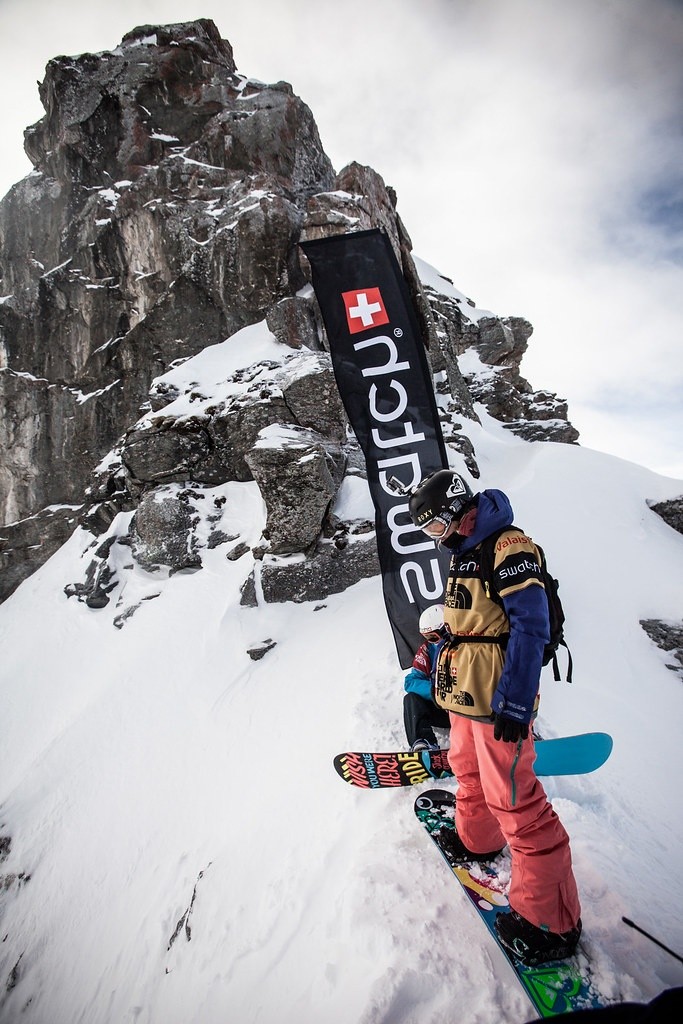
[422,629,443,644]
[419,516,450,539]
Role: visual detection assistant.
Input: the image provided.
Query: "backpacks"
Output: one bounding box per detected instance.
[448,525,565,666]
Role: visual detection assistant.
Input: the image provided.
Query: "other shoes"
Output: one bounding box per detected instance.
[437,825,503,863]
[413,742,439,751]
[494,908,582,966]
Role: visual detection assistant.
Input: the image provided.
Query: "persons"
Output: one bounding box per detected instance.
[408,470,583,952]
[403,604,451,751]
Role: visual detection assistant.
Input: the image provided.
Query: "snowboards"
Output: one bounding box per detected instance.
[414,788,626,1018]
[332,732,613,790]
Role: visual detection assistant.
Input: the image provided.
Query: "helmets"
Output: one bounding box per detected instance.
[409,470,474,527]
[419,604,451,634]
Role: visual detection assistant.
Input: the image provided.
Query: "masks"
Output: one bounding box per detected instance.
[441,524,467,549]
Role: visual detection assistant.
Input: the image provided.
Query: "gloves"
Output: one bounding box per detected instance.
[488,710,528,743]
